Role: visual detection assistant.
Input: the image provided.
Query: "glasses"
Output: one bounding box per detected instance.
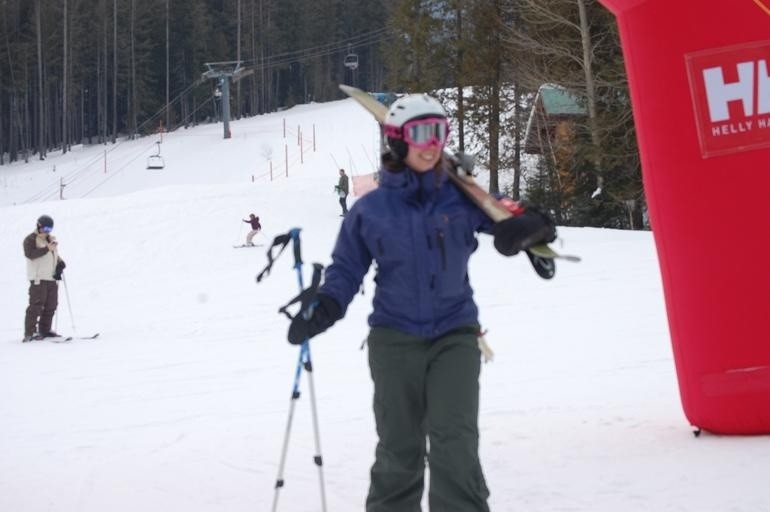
[41,225,53,232]
[384,118,451,148]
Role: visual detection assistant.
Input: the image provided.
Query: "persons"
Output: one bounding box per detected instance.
[23,215,66,342]
[334,168,349,215]
[288,92,558,512]
[243,213,262,246]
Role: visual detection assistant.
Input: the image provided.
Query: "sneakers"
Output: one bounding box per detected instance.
[24,335,44,342]
[39,332,57,337]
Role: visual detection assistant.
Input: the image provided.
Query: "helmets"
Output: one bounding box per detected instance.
[382,92,449,147]
[38,215,54,228]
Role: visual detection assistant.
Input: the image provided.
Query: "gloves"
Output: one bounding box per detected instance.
[287,293,345,346]
[53,262,64,280]
[490,208,559,257]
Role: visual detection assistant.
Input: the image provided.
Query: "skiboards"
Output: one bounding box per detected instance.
[338,82,582,280]
[21,332,99,342]
